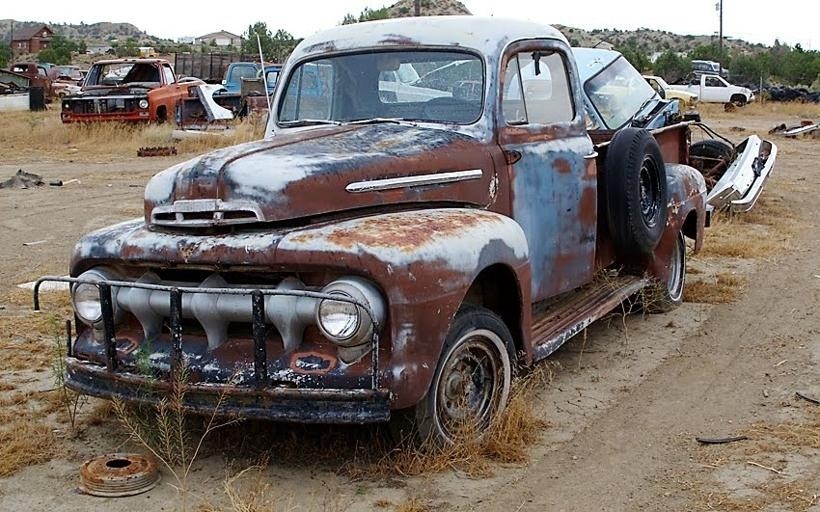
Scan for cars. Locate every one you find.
[0,46,539,123]
[593,75,700,110]
[690,60,728,81]
[32,11,715,459]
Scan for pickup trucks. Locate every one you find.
[664,73,756,108]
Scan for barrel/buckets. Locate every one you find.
[29,87,44,110]
